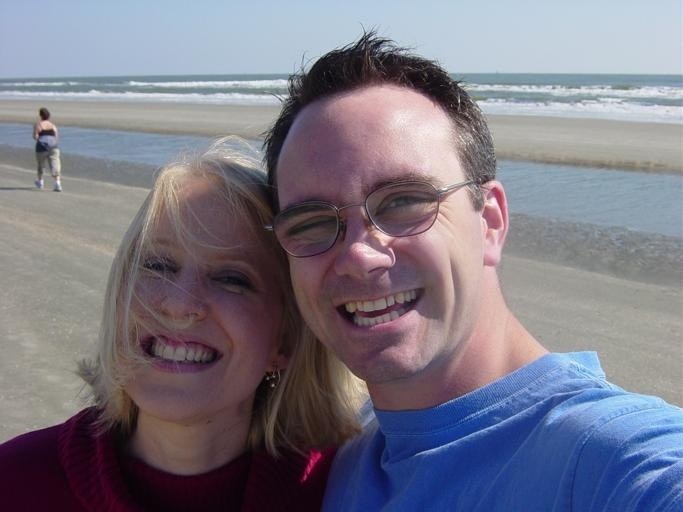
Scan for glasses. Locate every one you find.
[262,179,498,259]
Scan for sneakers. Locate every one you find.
[34,179,43,188]
[53,185,62,191]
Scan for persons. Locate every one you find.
[261,22,681,510]
[31,106,62,192]
[0,135,371,510]
